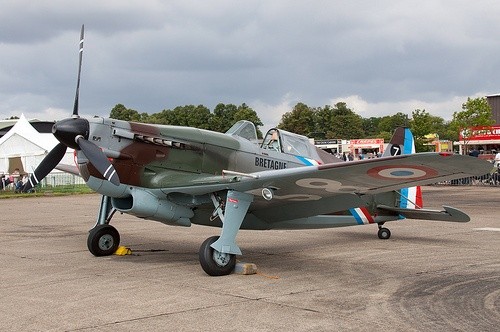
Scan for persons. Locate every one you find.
[0,168,20,190]
[330,151,382,161]
[16,172,28,189]
[469,147,478,157]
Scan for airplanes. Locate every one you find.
[20,23,497,276]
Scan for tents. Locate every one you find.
[0,113,66,174]
